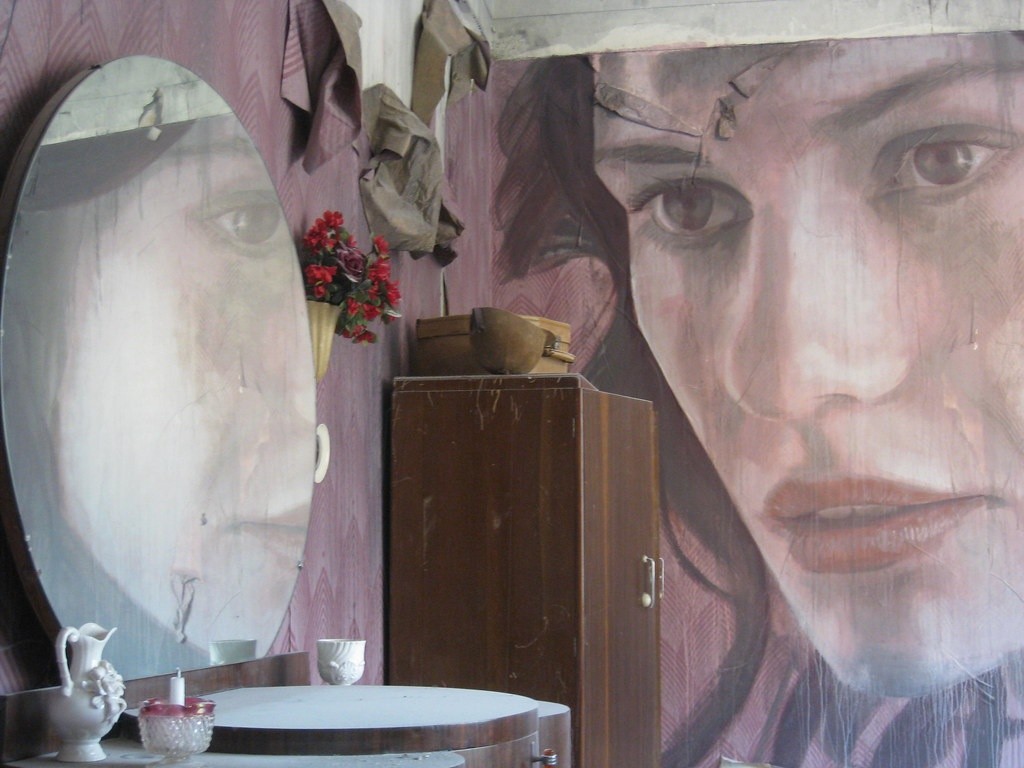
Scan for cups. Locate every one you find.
[138,697,216,766]
[316,638,366,686]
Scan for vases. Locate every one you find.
[306,301,343,380]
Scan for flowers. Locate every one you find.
[298,210,403,344]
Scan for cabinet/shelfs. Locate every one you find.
[389,376,663,768]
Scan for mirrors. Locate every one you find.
[0,55,316,681]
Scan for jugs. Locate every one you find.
[46,623,127,763]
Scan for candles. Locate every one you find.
[169,668,185,706]
